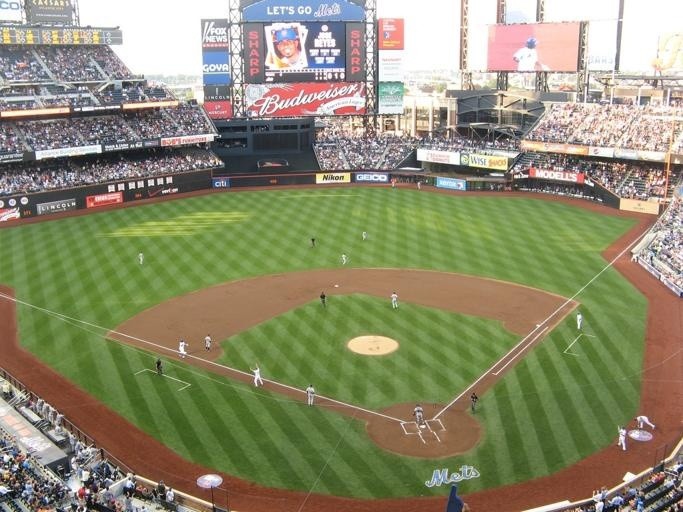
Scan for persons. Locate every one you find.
[616,424,626,452]
[155,357,163,375]
[0,375,174,512]
[313,127,520,191]
[137,251,142,264]
[272,28,301,67]
[311,238,315,247]
[563,451,682,512]
[203,333,212,353]
[411,402,424,425]
[340,253,346,264]
[178,340,188,359]
[390,292,398,311]
[0,44,226,218]
[250,363,263,388]
[447,485,470,512]
[304,384,316,408]
[513,36,548,70]
[519,65,682,296]
[318,290,325,306]
[576,311,582,330]
[361,231,367,241]
[633,416,655,431]
[470,393,477,411]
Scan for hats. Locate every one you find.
[272,28,299,42]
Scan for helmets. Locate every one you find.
[526,38,537,46]
